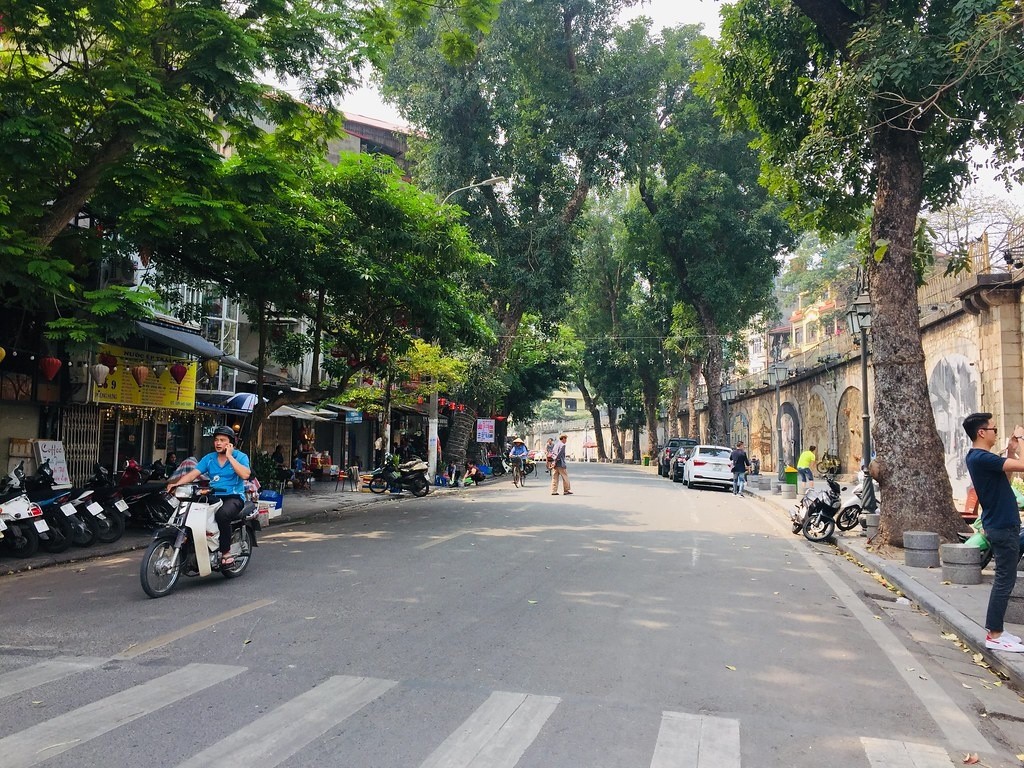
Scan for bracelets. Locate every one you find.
[1016,436,1024,440]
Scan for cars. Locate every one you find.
[682,445,734,489]
[669,445,694,482]
[528,450,547,461]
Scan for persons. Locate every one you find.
[167,426,251,565]
[446,461,459,480]
[730,440,750,498]
[166,452,177,470]
[545,438,555,473]
[797,446,816,488]
[962,413,1024,652]
[550,434,573,495]
[509,438,528,484]
[464,462,483,485]
[271,445,285,464]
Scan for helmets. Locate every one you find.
[213,425,235,441]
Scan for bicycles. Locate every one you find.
[509,454,525,488]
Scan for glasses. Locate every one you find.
[981,428,997,433]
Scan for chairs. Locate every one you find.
[335,467,357,491]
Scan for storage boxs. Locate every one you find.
[257,500,277,519]
[322,465,339,476]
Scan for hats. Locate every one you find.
[513,438,524,443]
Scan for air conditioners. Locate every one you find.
[106,255,138,287]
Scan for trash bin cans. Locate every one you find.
[785,465,798,493]
[644,455,649,466]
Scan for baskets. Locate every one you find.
[547,457,554,462]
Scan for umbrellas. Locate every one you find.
[227,392,268,438]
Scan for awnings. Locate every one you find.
[111,315,290,382]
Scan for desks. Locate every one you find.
[294,471,314,489]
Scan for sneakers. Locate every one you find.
[1002,630,1022,643]
[985,634,1024,652]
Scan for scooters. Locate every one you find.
[488,451,534,477]
[963,485,1024,570]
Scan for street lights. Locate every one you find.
[584,420,589,462]
[719,380,736,448]
[659,409,668,446]
[766,361,789,491]
[845,285,871,536]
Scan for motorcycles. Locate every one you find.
[369,452,433,497]
[837,466,881,531]
[0,458,173,559]
[790,474,847,542]
[140,484,262,598]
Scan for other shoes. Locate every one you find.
[521,471,524,475]
[552,493,559,495]
[545,469,549,473]
[564,492,572,495]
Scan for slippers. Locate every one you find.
[221,556,234,566]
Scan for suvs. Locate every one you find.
[658,438,699,477]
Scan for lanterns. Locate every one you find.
[91,363,110,406]
[169,364,187,400]
[0,347,6,361]
[132,365,148,388]
[203,359,218,395]
[41,355,62,404]
